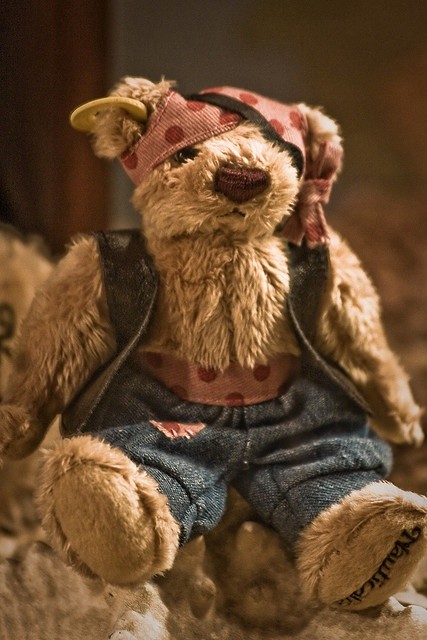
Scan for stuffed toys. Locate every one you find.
[1,76,427,613]
[2,224,427,639]
